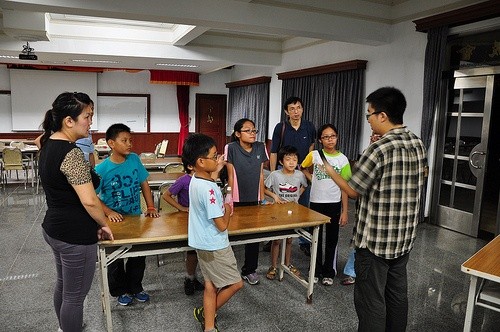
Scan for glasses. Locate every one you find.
[321,135,337,140]
[366,111,389,120]
[241,129,257,134]
[197,152,218,166]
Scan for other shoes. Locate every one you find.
[308,276,319,283]
[287,264,301,277]
[267,266,278,279]
[322,278,333,285]
[202,326,220,332]
[194,306,218,326]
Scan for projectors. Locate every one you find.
[19,54,38,60]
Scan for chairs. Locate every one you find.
[157,182,178,266]
[139,189,155,214]
[0,147,31,190]
[10,141,38,184]
[163,160,185,173]
[0,139,10,190]
[156,139,169,158]
[141,153,157,159]
[96,137,108,145]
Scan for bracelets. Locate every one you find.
[147,207,155,209]
[262,199,267,204]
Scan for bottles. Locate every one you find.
[225,187,234,216]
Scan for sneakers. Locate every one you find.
[134,291,150,302]
[118,294,133,306]
[192,276,204,291]
[184,276,195,296]
[242,273,259,285]
[342,274,355,284]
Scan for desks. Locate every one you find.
[139,172,225,187]
[96,202,330,332]
[459,235,500,332]
[0,138,43,194]
[94,157,185,169]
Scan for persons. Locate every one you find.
[325,87,430,332]
[162,157,206,296]
[263,96,316,256]
[263,145,308,281]
[223,118,274,285]
[92,124,160,305]
[38,92,113,332]
[300,124,351,284]
[182,133,246,332]
[344,132,382,284]
